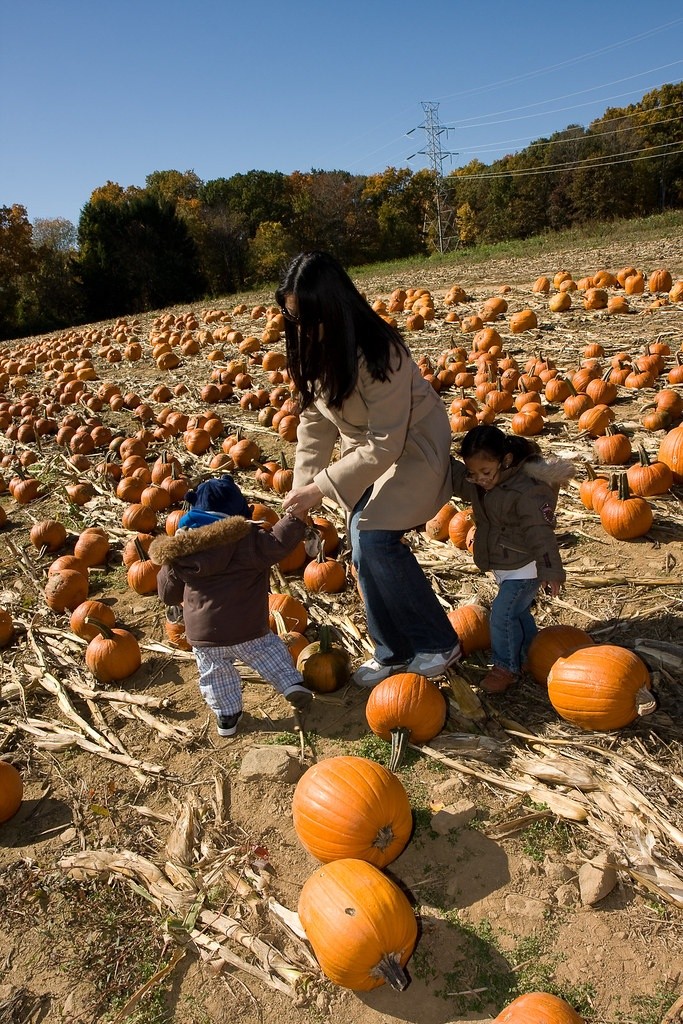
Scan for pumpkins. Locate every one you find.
[296,858,419,994]
[360,268,683,772]
[292,754,413,869]
[0,305,349,691]
[491,992,584,1024]
[0,761,23,823]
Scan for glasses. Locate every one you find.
[463,462,501,485]
[281,309,300,324]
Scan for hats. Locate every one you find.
[186,475,250,518]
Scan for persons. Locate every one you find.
[275,251,463,690]
[453,425,572,694]
[148,474,313,737]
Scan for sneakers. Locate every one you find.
[284,684,314,708]
[480,666,519,693]
[353,658,419,687]
[217,710,244,736]
[407,643,462,678]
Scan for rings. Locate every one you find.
[289,506,295,513]
